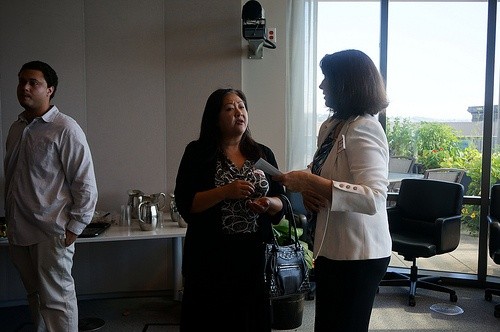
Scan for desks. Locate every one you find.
[0,212,188,302]
[388,173,424,181]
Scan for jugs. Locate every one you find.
[169,193,180,222]
[127,189,145,220]
[145,191,166,224]
[137,201,159,231]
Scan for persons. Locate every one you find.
[5,61,98,332]
[272,50,392,332]
[174,88,287,332]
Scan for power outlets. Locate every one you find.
[268,27,276,42]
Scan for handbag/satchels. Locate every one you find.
[256,193,315,306]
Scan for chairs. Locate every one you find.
[376,179,464,307]
[285,187,316,300]
[390,156,464,183]
[484,184,500,317]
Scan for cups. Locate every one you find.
[179,215,188,228]
[119,205,130,226]
